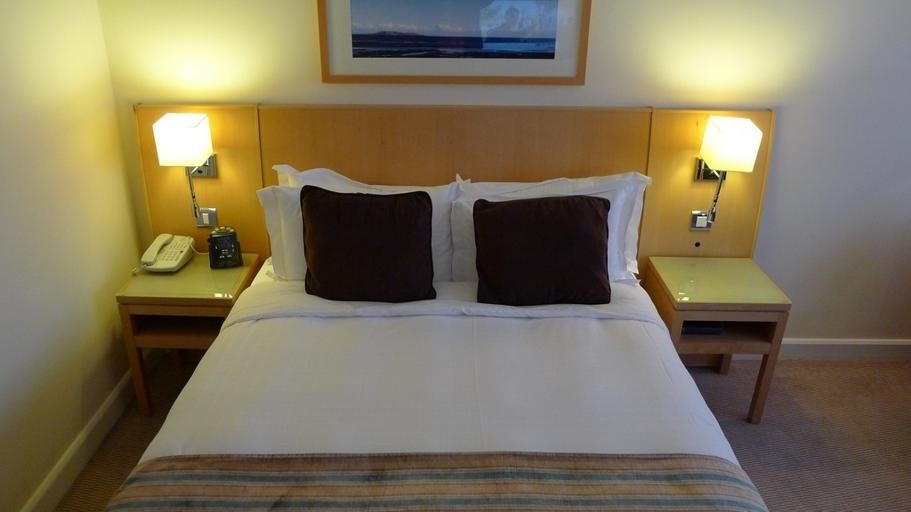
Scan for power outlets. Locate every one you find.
[689,211,714,231]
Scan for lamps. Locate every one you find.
[152,113,215,224]
[694,114,763,227]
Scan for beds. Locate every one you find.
[104,104,770,511]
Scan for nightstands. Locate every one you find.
[115,250,259,418]
[645,253,791,427]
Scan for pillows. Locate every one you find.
[255,181,455,281]
[271,163,473,198]
[450,173,650,287]
[471,195,615,306]
[299,183,438,306]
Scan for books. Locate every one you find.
[683,323,721,335]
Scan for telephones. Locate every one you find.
[140,234,195,273]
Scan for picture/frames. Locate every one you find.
[317,0,593,87]
